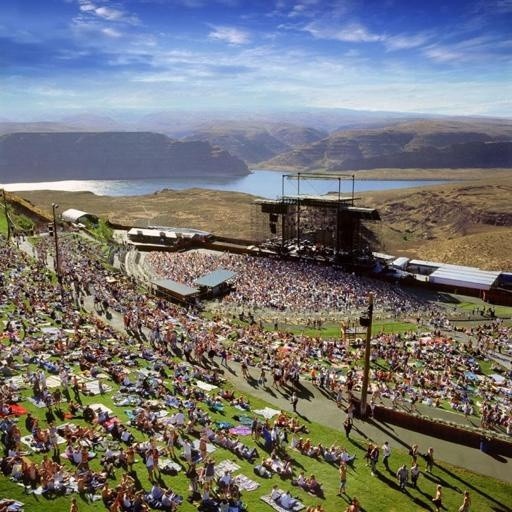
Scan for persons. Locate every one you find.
[1,225,511,512]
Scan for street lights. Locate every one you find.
[359,291,373,415]
[48,203,59,271]
[0,189,7,209]
